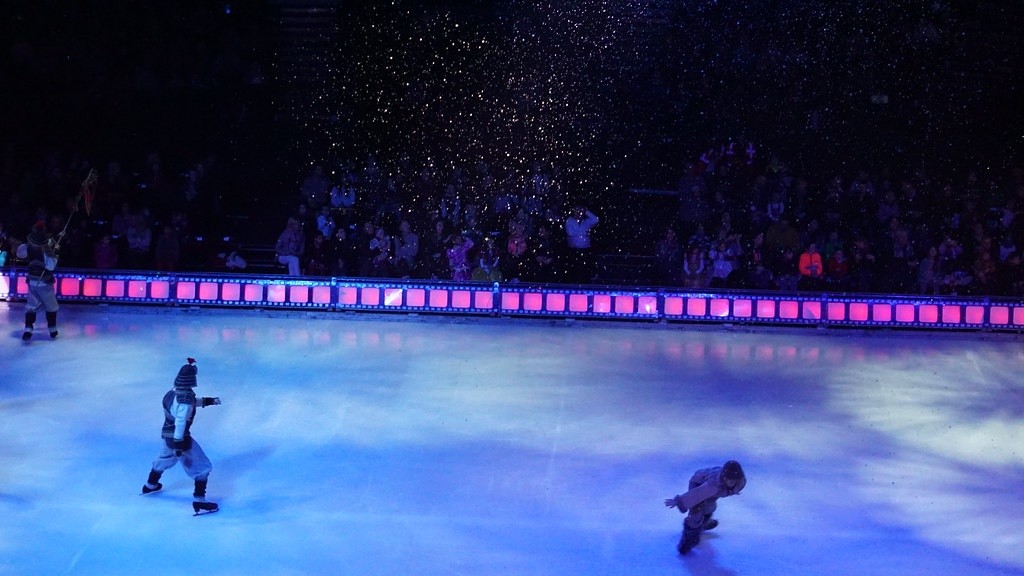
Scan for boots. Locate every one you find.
[44,311,59,341]
[22,312,36,343]
[193,479,219,516]
[138,466,164,496]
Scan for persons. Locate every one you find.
[141,363,221,518]
[273,142,602,284]
[20,220,59,339]
[651,125,1023,295]
[665,460,748,554]
[0,161,248,270]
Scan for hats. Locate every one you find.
[174,357,198,389]
[719,460,745,480]
[26,220,50,248]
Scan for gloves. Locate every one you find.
[174,439,184,458]
[202,396,221,409]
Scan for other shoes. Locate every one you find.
[677,514,719,555]
